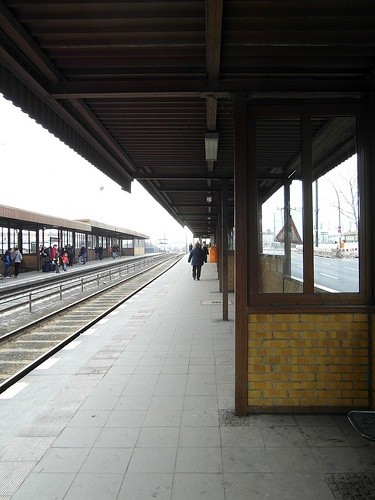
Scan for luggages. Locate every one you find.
[42,264,49,272]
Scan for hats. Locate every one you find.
[64,253,68,255]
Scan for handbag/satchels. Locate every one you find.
[1,255,7,262]
[48,261,55,271]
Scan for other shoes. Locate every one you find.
[6,276,11,278]
[15,275,19,278]
[56,271,60,273]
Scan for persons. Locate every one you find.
[11,248,23,278]
[1,248,12,278]
[40,245,76,273]
[78,247,87,265]
[95,246,103,260]
[189,241,209,263]
[112,244,121,259]
[188,243,207,280]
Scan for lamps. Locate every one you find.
[207,206,212,232]
[204,130,219,162]
[206,194,213,203]
